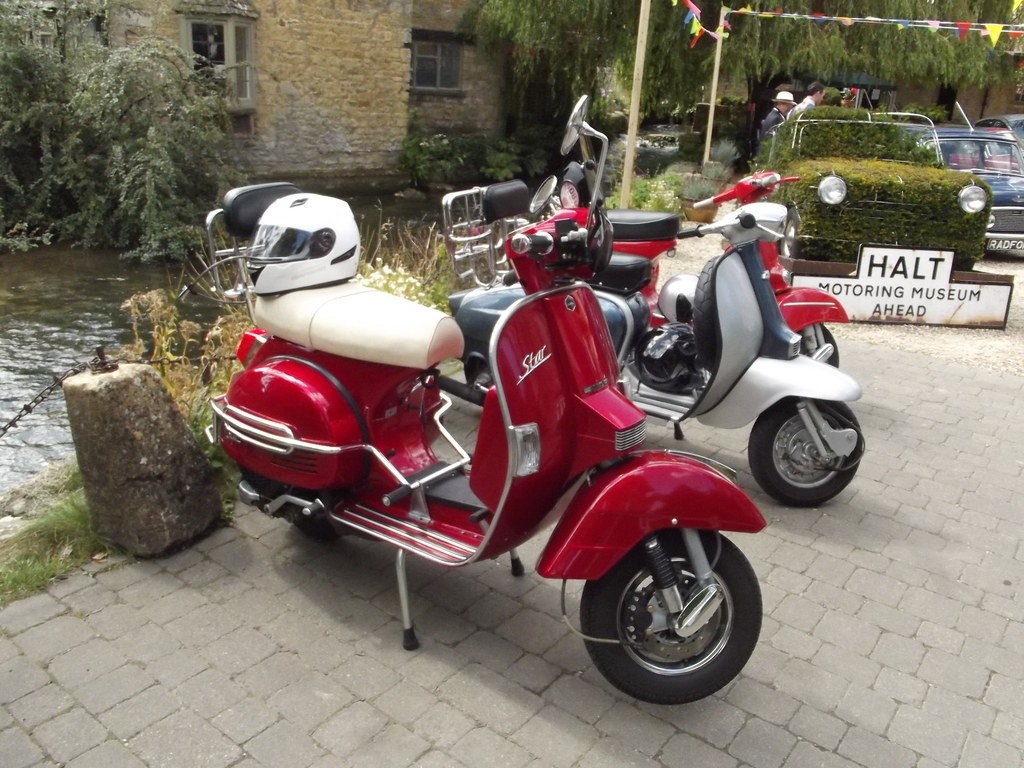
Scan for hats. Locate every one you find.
[771,91,797,106]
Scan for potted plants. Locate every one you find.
[701,160,733,192]
[680,164,728,223]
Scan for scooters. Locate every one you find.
[200,93,769,706]
[440,179,866,509]
[598,156,852,371]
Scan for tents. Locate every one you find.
[801,69,900,117]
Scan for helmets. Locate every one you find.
[631,322,705,394]
[659,272,697,322]
[245,192,362,295]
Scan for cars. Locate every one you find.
[948,114,1024,172]
[899,125,1023,251]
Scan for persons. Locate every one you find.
[760,81,826,144]
